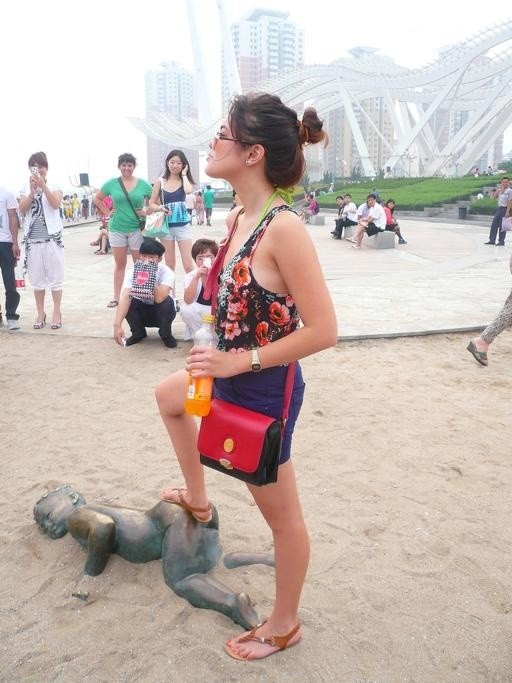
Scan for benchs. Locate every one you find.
[309,213,325,225]
[343,225,396,249]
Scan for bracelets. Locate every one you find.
[181,173,187,177]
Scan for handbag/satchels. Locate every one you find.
[500,216,512,233]
[141,210,170,238]
[129,259,158,306]
[138,221,200,230]
[197,397,283,488]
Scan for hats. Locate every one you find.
[139,240,166,256]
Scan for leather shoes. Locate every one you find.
[157,328,178,349]
[346,237,358,243]
[125,332,148,346]
[351,245,361,249]
[331,230,341,239]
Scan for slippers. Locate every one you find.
[95,248,106,255]
[90,240,99,246]
[107,300,118,307]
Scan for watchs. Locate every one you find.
[250,348,263,374]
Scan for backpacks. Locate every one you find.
[311,201,320,216]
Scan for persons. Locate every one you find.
[476,190,484,199]
[93,152,162,308]
[59,191,112,223]
[300,184,408,249]
[0,183,22,332]
[112,238,178,349]
[233,187,242,207]
[146,148,197,312]
[177,238,220,343]
[14,149,65,330]
[202,184,214,227]
[486,164,493,174]
[482,176,512,246]
[195,190,205,225]
[89,214,109,255]
[465,250,511,366]
[470,167,480,177]
[152,89,339,666]
[491,188,498,198]
[33,482,278,631]
[483,190,490,199]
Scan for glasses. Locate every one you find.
[213,128,255,146]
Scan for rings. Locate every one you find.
[38,174,41,177]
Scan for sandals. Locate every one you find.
[466,340,489,367]
[50,312,61,329]
[223,618,303,661]
[160,487,214,524]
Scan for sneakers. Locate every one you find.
[496,240,505,246]
[484,241,495,245]
[7,319,21,330]
[197,220,211,226]
[398,238,407,244]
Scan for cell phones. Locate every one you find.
[31,167,40,179]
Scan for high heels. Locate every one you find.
[33,312,46,329]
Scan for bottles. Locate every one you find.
[183,314,216,418]
